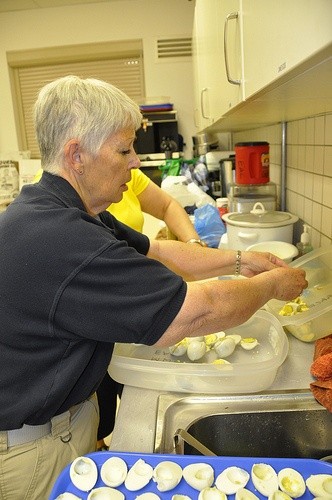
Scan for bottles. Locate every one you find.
[165,143,171,161]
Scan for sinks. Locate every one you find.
[152,388,332,467]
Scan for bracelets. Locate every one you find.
[233,250,241,277]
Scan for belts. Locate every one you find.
[6,404,79,448]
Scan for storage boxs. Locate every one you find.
[142,110,178,120]
[107,309,291,397]
[263,245,332,343]
[132,120,180,156]
[139,103,173,112]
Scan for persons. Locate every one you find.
[0,75,308,500]
[25,166,208,453]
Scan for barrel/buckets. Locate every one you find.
[234,142,271,185]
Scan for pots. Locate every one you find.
[221,201,299,250]
[192,132,220,160]
[219,154,236,197]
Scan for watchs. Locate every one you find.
[187,239,208,247]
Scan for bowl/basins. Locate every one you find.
[244,240,299,263]
[107,309,289,395]
[265,244,332,343]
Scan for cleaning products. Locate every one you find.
[294,223,313,260]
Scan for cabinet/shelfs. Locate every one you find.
[188,0,332,135]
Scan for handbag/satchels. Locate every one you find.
[194,205,226,247]
[160,175,216,210]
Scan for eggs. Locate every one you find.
[54,456,332,500]
[168,331,258,371]
[279,297,315,340]
